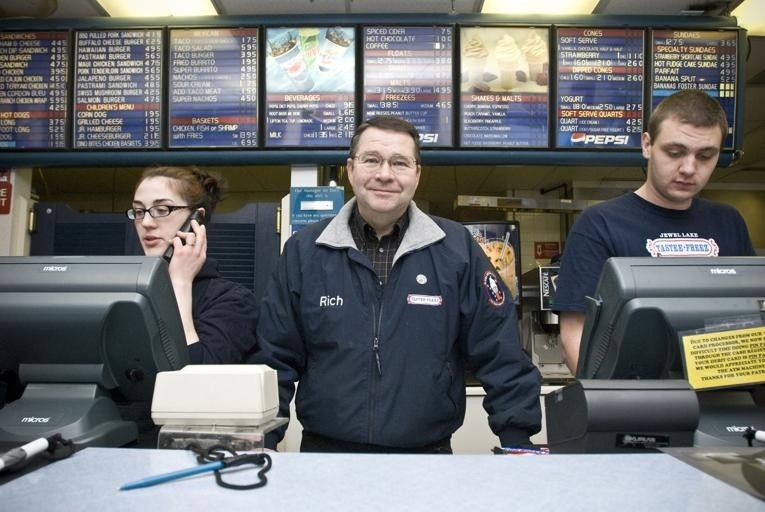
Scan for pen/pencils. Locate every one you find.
[119,460,226,492]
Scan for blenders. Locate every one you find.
[522,267,566,386]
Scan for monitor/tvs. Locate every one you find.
[574,256,761,451]
[2,256,193,449]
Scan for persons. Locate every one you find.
[129,165,259,369]
[250,115,545,456]
[551,91,757,387]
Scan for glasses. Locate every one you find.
[350,151,418,176]
[125,204,191,222]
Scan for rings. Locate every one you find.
[186,243,196,246]
